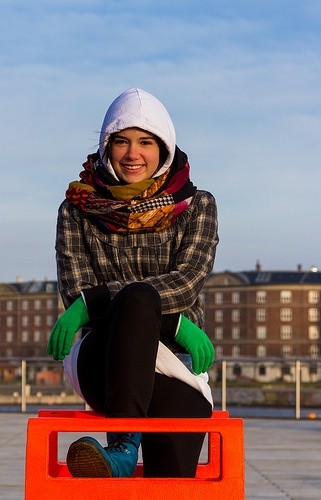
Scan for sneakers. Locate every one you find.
[66,436,138,478]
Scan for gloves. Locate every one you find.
[47,295,89,361]
[174,314,214,375]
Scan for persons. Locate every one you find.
[47,88,219,477]
